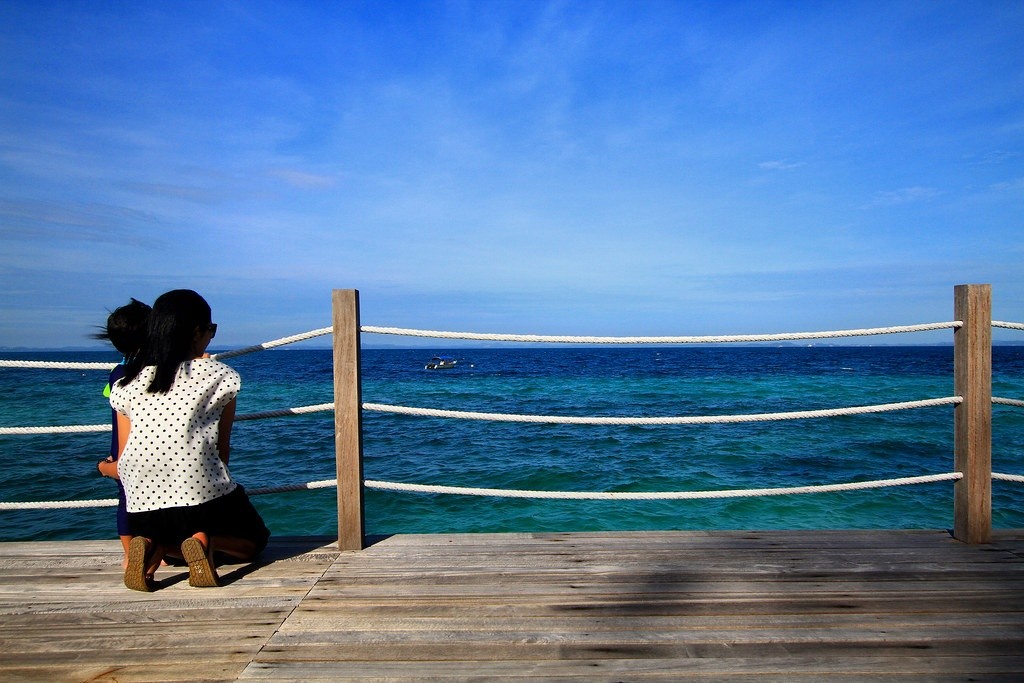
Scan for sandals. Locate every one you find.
[181,532,223,588]
[124,537,156,592]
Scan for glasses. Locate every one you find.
[208,322,218,340]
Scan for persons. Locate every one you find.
[97,289,270,592]
[87,298,210,570]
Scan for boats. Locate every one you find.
[424,356,458,369]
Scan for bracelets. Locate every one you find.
[97,458,112,478]
